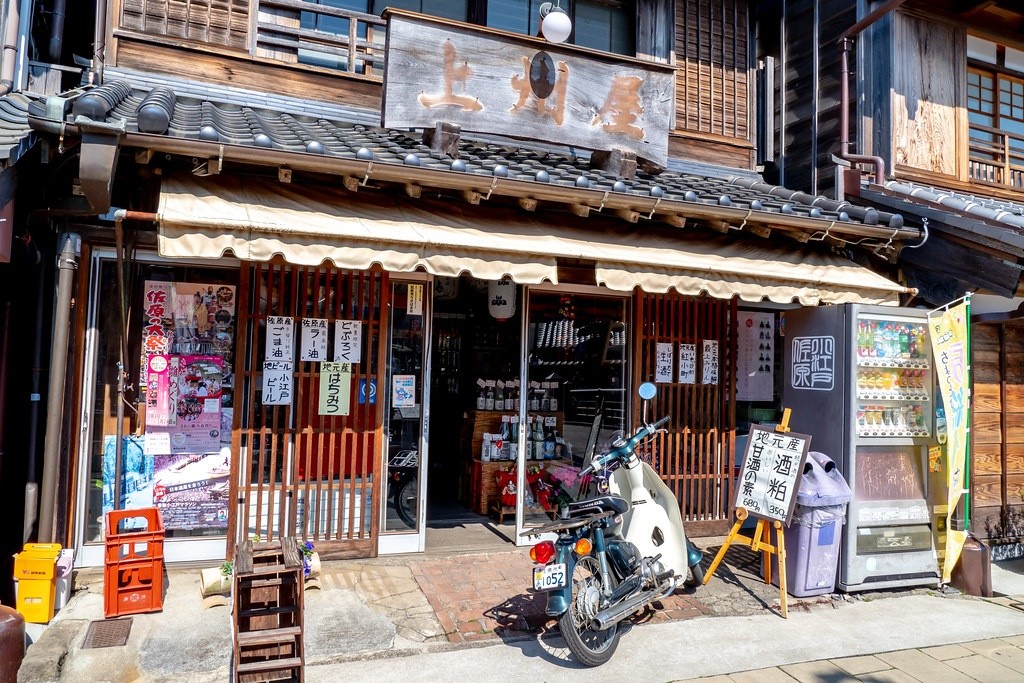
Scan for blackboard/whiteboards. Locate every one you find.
[732,424,812,527]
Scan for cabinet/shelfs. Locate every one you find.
[466,407,575,515]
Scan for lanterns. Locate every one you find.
[487,279,517,321]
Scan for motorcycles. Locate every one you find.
[389,450,417,526]
[518,382,704,669]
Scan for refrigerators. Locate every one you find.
[782,301,946,594]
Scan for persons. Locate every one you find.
[185,380,207,396]
[190,286,231,333]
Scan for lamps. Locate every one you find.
[538,0,571,42]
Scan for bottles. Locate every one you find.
[906,404,916,427]
[860,320,928,391]
[474,379,564,461]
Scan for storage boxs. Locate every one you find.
[102,506,165,618]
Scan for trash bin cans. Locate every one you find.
[760,450,853,597]
